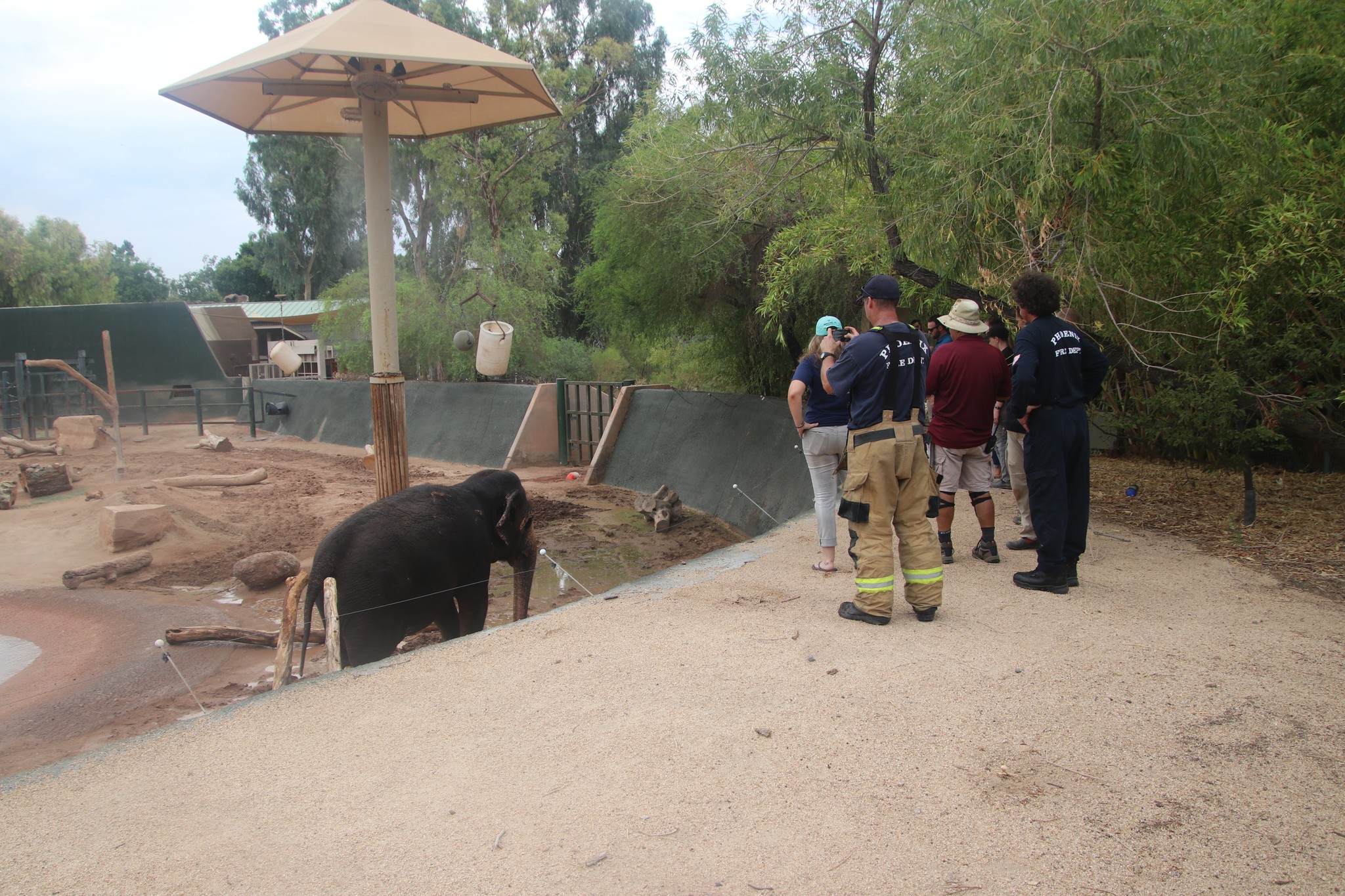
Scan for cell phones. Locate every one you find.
[832,329,851,342]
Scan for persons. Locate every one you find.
[908,313,953,480]
[1009,270,1108,595]
[818,273,944,625]
[1056,304,1081,327]
[926,298,1009,565]
[978,307,1038,551]
[786,315,859,573]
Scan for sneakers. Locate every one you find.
[972,538,1000,563]
[938,538,954,564]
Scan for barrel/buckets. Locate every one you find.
[476,321,514,376]
[269,341,302,374]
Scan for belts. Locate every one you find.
[853,424,924,447]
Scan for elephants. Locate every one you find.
[298,468,536,679]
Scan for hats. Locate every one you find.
[856,273,900,301]
[816,315,842,336]
[937,298,989,333]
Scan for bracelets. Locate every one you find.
[795,421,804,428]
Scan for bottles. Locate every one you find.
[1126,484,1139,497]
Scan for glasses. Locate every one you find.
[928,325,940,332]
[861,286,873,298]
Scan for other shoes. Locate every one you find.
[1006,537,1043,550]
[990,479,1012,489]
[1012,511,1022,524]
[838,602,891,625]
[912,606,938,620]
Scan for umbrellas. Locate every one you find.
[154,0,559,498]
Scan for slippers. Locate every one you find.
[812,561,838,572]
[993,475,1002,479]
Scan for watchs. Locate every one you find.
[994,405,1002,410]
[820,352,835,361]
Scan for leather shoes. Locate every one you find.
[1013,564,1069,594]
[1067,557,1079,586]
